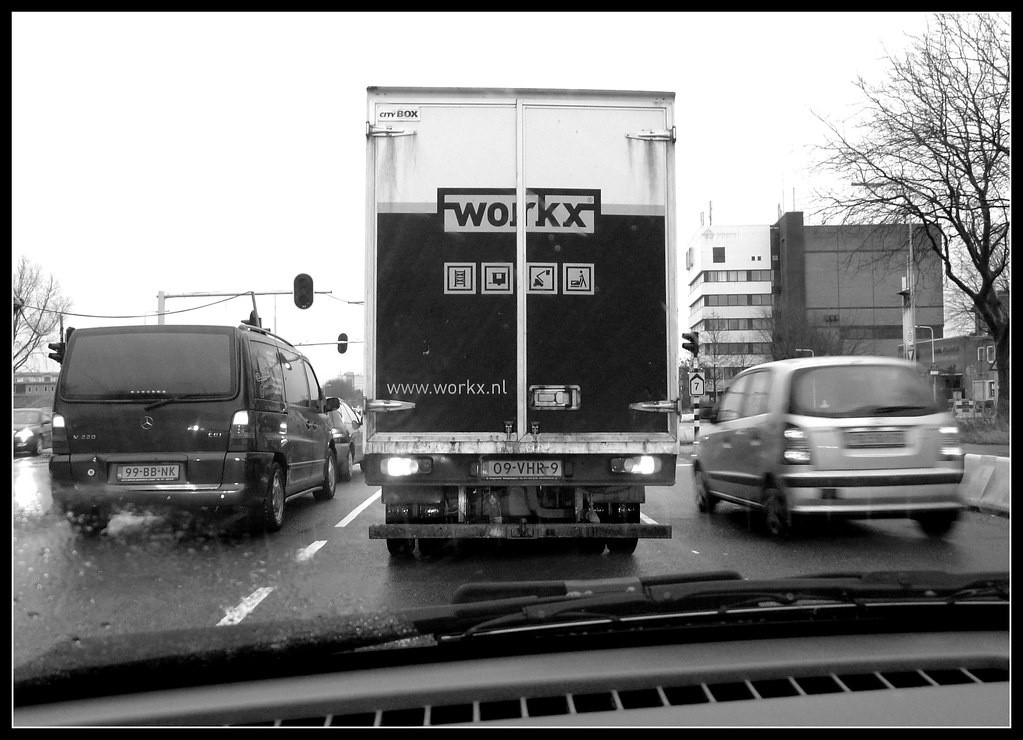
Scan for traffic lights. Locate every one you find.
[681,330,701,356]
[242,311,258,332]
[293,274,314,310]
[48,340,66,366]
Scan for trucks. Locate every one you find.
[359,84,680,562]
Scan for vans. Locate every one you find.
[48,324,342,537]
[691,356,968,541]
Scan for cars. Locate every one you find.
[11,408,54,456]
[324,398,364,483]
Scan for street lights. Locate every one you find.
[915,324,936,404]
[853,181,916,362]
[796,348,816,409]
